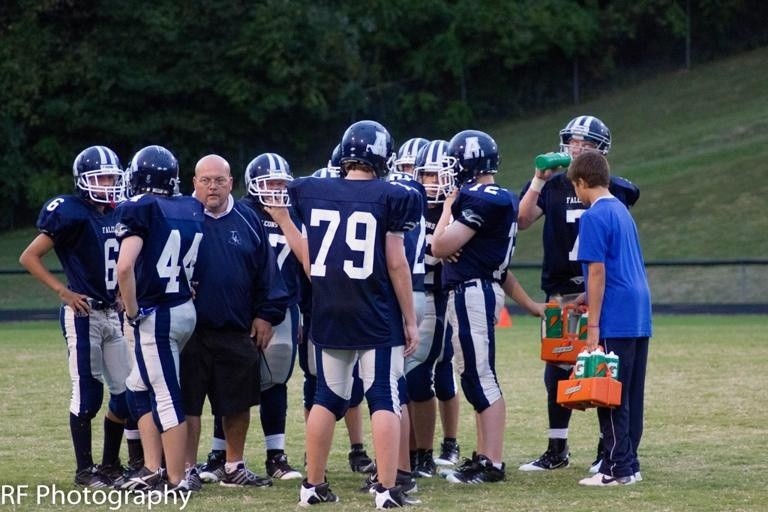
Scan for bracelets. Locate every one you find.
[586,324,600,327]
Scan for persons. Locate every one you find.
[514,113,640,475]
[565,151,654,488]
[16,119,554,509]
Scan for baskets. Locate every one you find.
[541,305,623,411]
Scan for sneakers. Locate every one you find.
[74,442,643,510]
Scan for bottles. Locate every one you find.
[533,150,574,173]
[540,299,588,339]
[573,348,620,378]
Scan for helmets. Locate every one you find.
[73,115,612,206]
